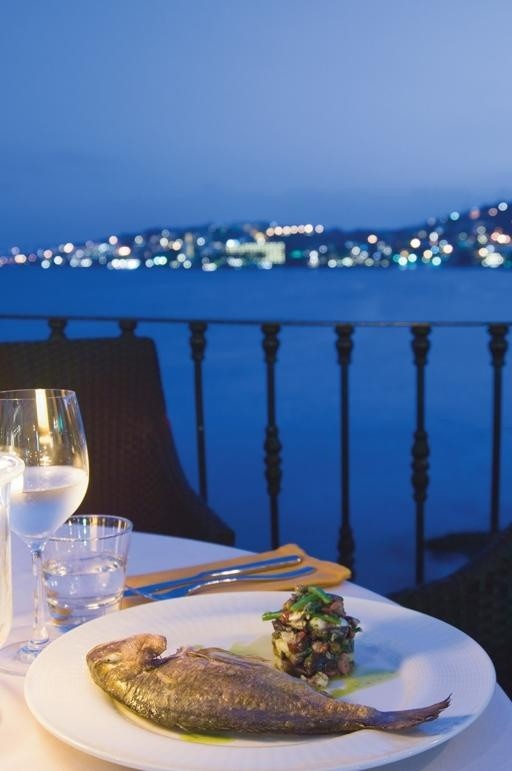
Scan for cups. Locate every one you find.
[39,515,133,633]
[0,389,90,671]
[0,453,27,650]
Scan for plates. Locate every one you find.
[22,591,496,766]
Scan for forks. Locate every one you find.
[131,566,316,602]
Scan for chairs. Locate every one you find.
[388,518,512,696]
[0,337,235,546]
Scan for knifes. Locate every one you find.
[124,553,301,598]
